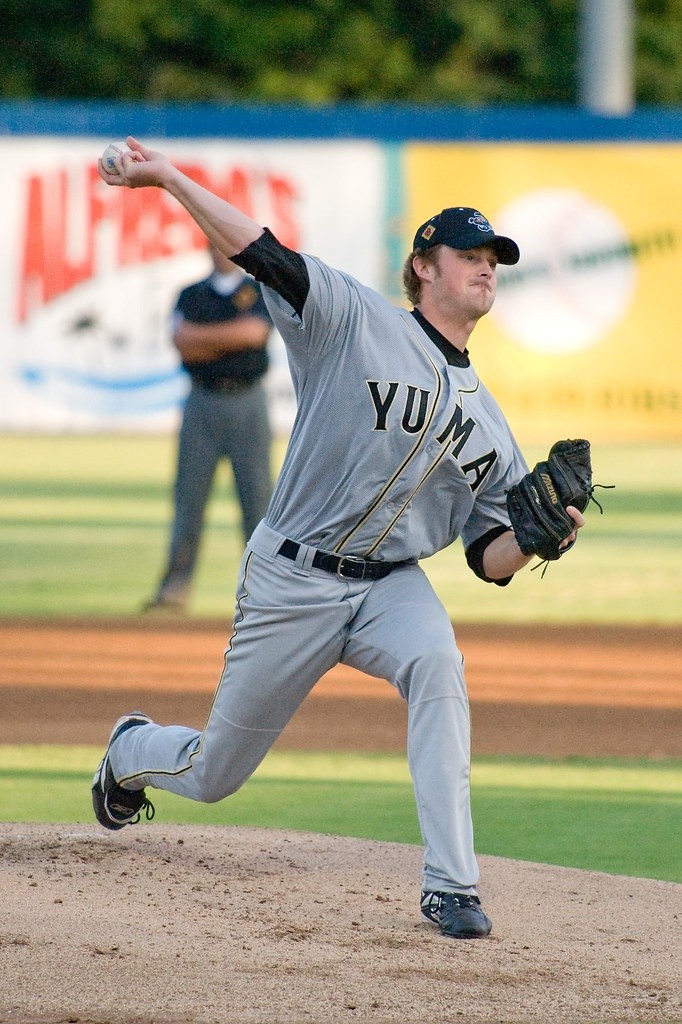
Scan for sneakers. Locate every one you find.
[418,891,493,938]
[91,712,153,830]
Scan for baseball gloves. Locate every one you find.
[505,438,593,561]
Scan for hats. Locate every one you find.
[414,207,521,266]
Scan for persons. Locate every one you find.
[142,238,273,611]
[91,136,595,938]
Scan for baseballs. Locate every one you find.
[101,142,131,175]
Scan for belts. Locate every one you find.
[279,542,394,582]
